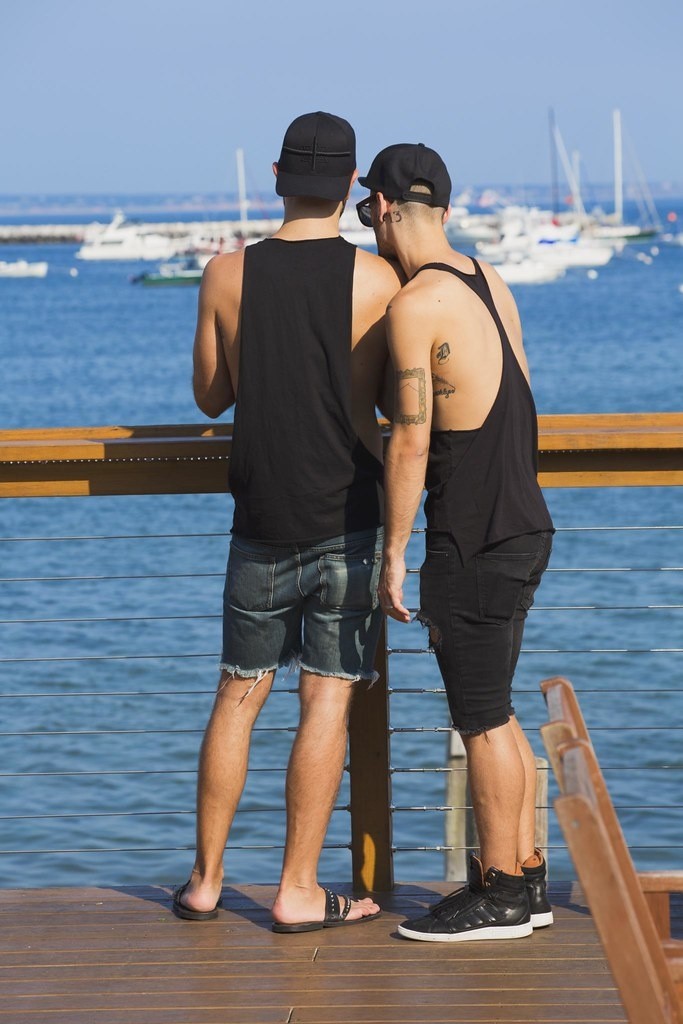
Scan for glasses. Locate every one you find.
[356,194,381,227]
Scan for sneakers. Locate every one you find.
[520,847,554,928]
[397,856,533,942]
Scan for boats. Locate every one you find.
[71,108,660,283]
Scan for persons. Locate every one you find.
[353,141,555,943]
[170,112,404,934]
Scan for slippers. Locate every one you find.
[172,880,219,920]
[272,886,383,932]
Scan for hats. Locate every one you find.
[276,111,357,200]
[357,142,452,208]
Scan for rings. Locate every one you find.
[386,606,393,609]
